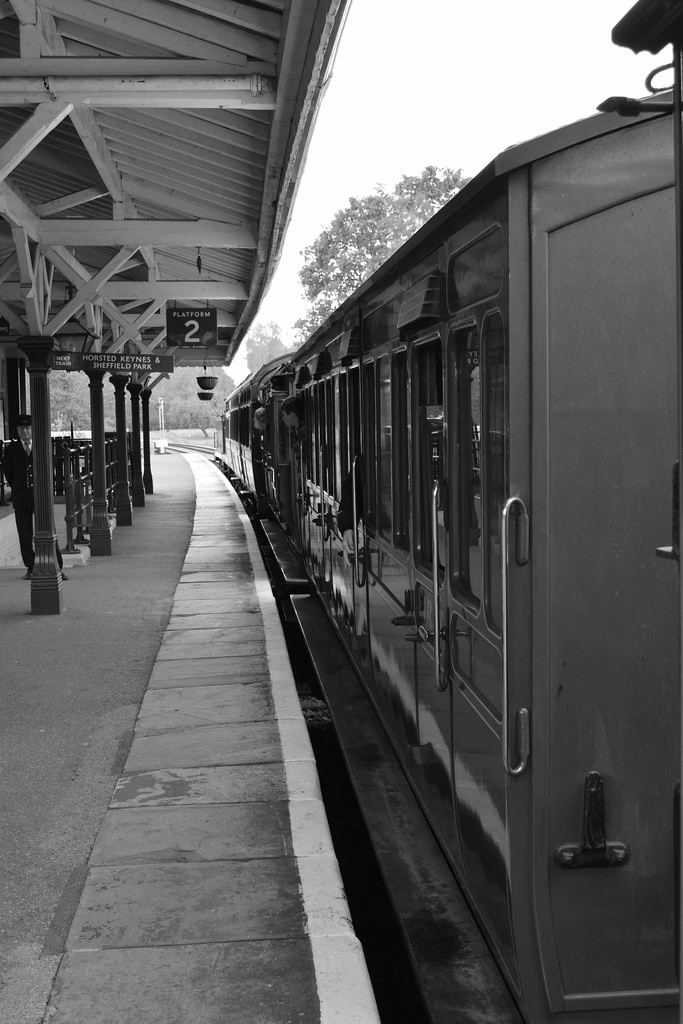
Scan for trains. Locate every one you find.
[215,86,683,1024]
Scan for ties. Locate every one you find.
[24,442,31,456]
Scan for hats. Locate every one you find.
[15,415,32,426]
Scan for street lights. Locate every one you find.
[601,1,683,992]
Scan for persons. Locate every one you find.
[1,415,68,580]
[280,396,304,429]
[253,407,269,430]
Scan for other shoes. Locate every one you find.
[60,572,68,580]
[24,571,33,580]
